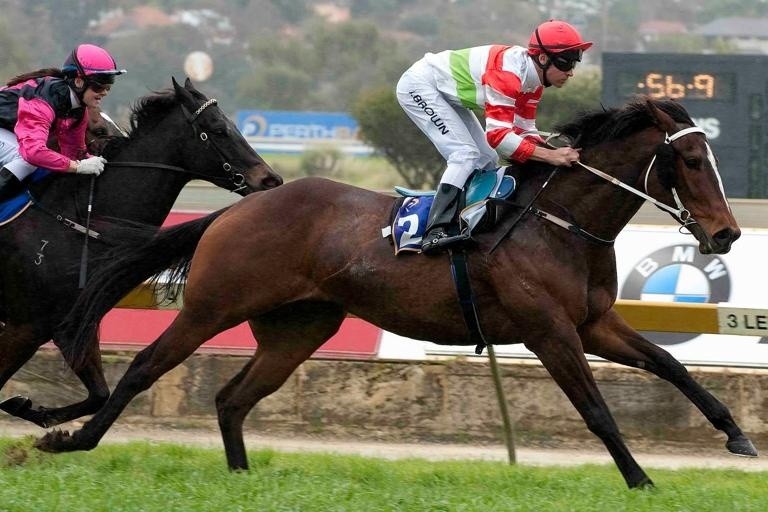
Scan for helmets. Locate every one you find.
[528,19,593,57]
[61,44,127,77]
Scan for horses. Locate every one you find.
[0,77,283,428]
[31,95,759,495]
[88,107,129,155]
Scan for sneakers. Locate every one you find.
[421,182,469,255]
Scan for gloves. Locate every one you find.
[77,155,107,176]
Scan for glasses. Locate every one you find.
[545,52,576,71]
[88,80,111,92]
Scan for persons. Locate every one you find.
[395,19,592,250]
[1,43,125,195]
